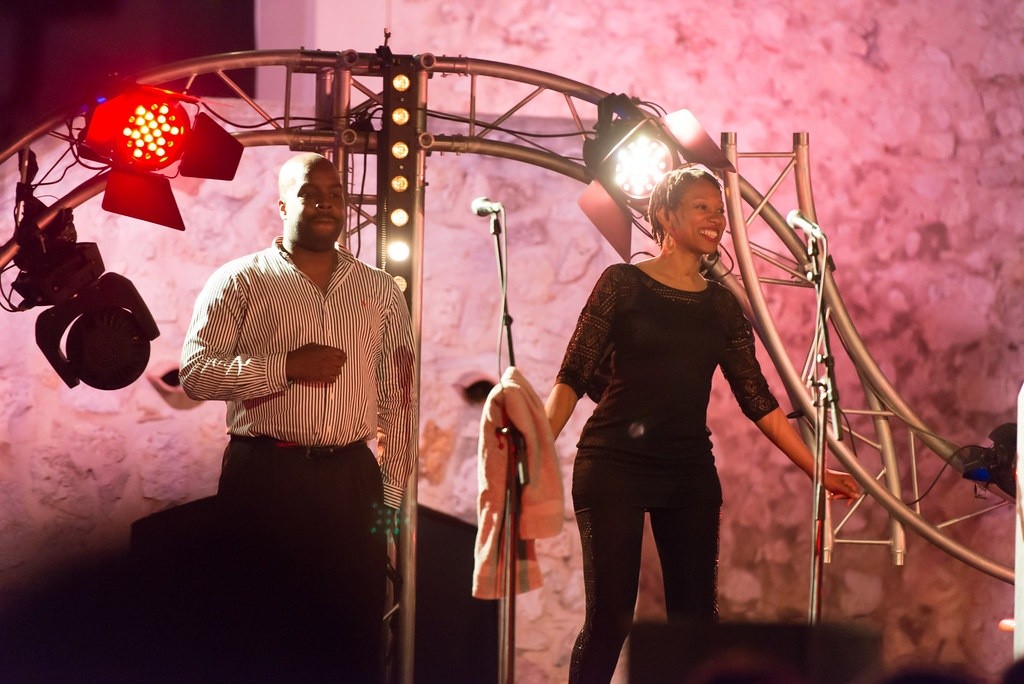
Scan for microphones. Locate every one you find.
[787,209,827,240]
[471,196,502,217]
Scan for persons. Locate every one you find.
[179,153,420,683]
[542,165,861,684]
